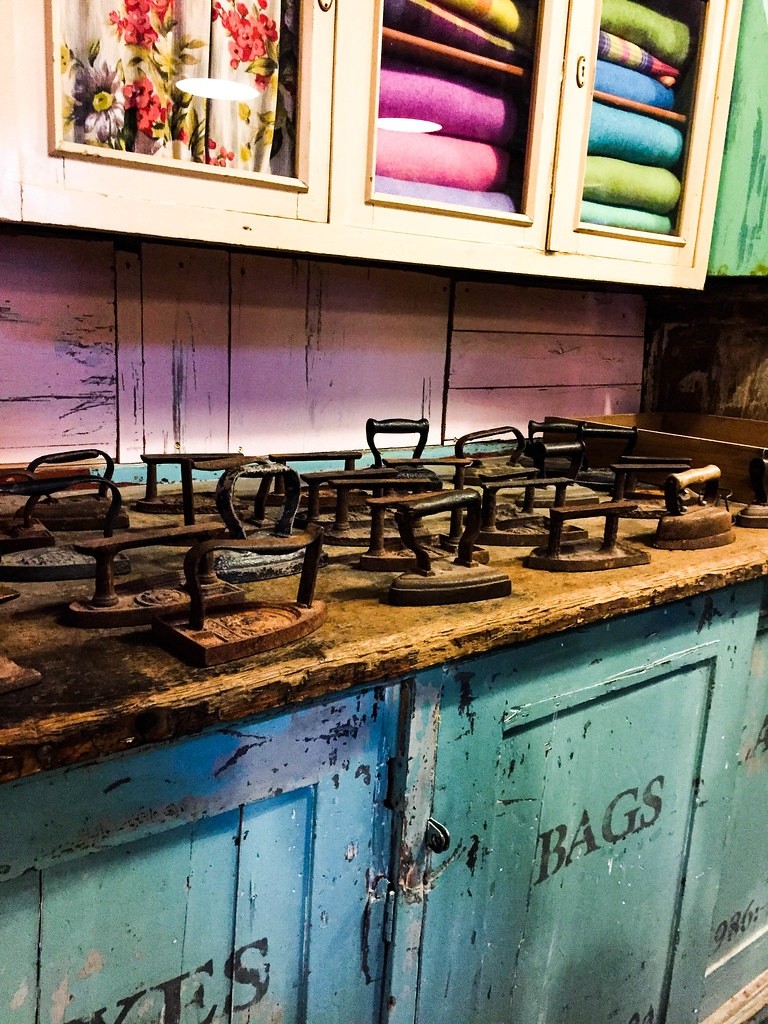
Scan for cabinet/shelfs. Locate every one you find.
[3,574,768,1024]
[0,0,744,292]
